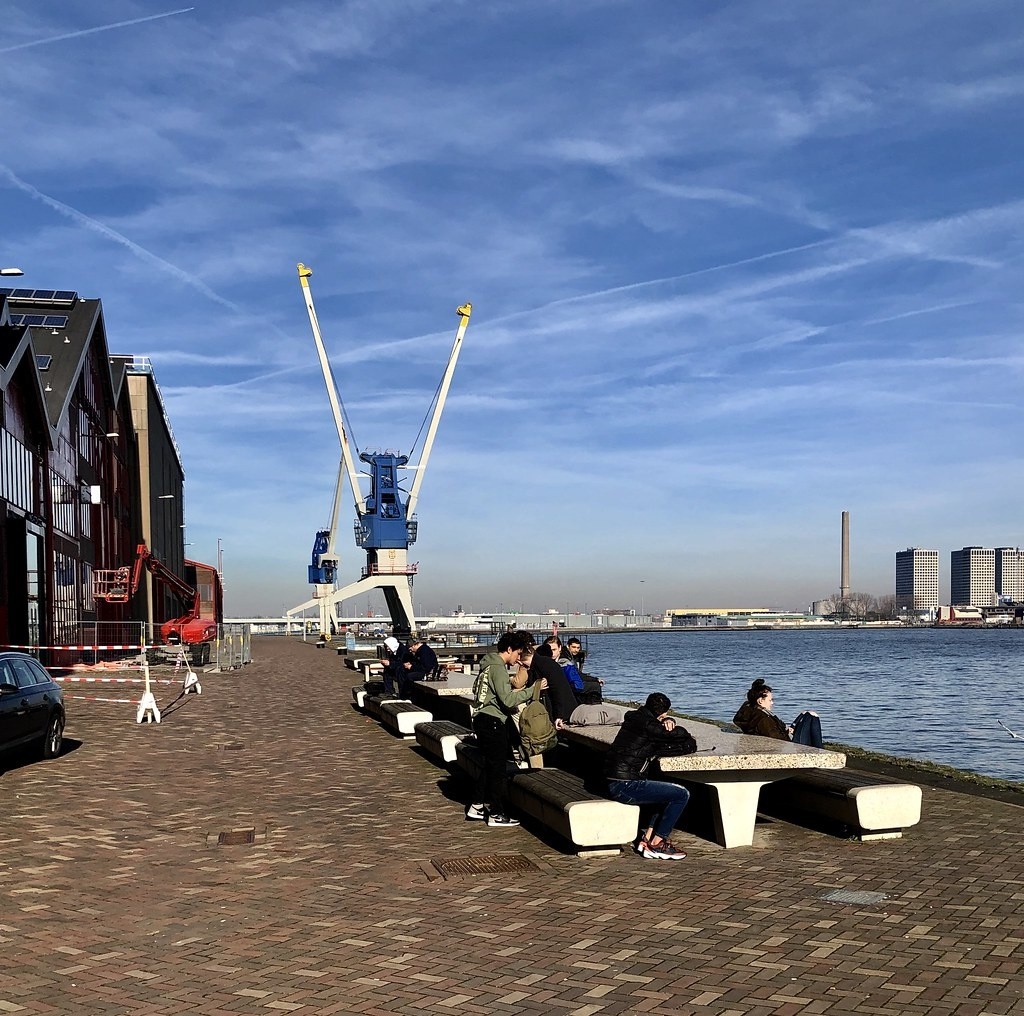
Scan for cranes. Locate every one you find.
[282,261,473,639]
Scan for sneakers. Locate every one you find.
[488,813,520,826]
[467,804,489,820]
[637,835,648,852]
[643,837,687,860]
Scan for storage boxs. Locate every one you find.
[308,565,337,584]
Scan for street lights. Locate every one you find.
[217,538,223,572]
[220,549,224,584]
[640,581,646,615]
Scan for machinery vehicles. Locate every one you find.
[90,543,218,667]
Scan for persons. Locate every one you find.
[466,630,604,827]
[392,637,439,700]
[604,692,689,859]
[379,637,414,696]
[732,678,823,749]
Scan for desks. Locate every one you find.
[378,670,478,714]
[456,693,847,849]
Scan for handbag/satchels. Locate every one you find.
[570,674,604,705]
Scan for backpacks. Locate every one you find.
[427,664,449,681]
[519,679,558,757]
[791,710,825,749]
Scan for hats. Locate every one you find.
[406,639,419,650]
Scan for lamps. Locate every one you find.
[154,494,175,500]
[183,543,196,546]
[173,525,188,528]
[81,432,119,438]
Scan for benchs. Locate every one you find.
[770,770,922,842]
[414,721,476,763]
[514,768,641,859]
[352,685,369,710]
[380,702,432,740]
[367,694,412,722]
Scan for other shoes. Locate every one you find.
[379,692,393,700]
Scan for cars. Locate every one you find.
[0,650,67,761]
